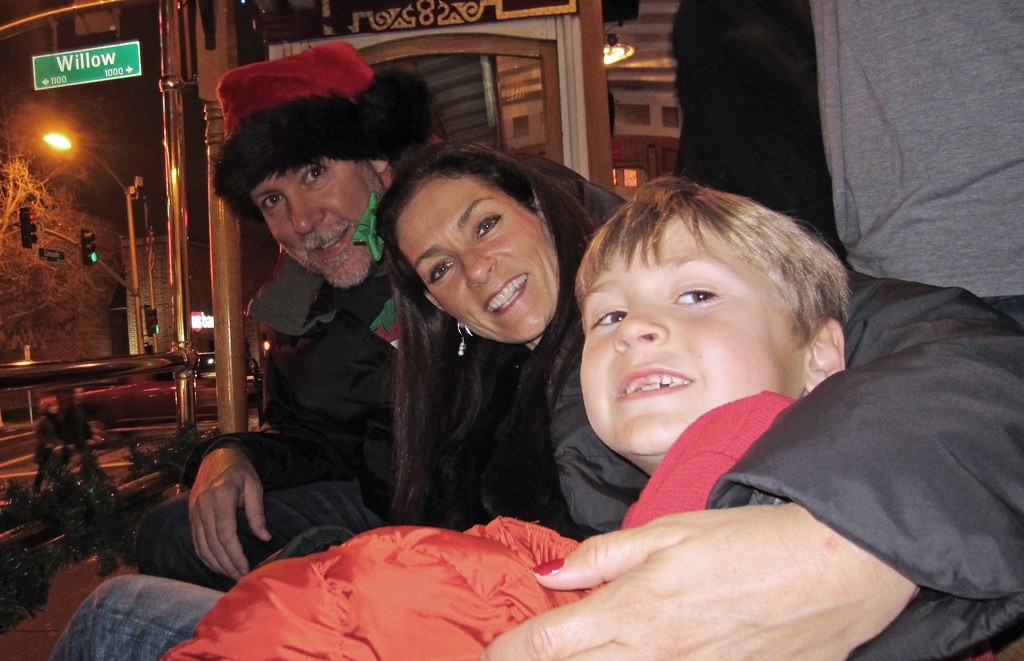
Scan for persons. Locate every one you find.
[32,397,76,494]
[51,0,1024,661]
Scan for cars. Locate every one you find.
[36,346,267,449]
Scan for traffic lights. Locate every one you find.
[144,304,159,337]
[81,227,98,267]
[19,207,38,249]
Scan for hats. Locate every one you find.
[39,396,57,413]
[212,42,429,223]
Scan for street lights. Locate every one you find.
[43,125,145,355]
